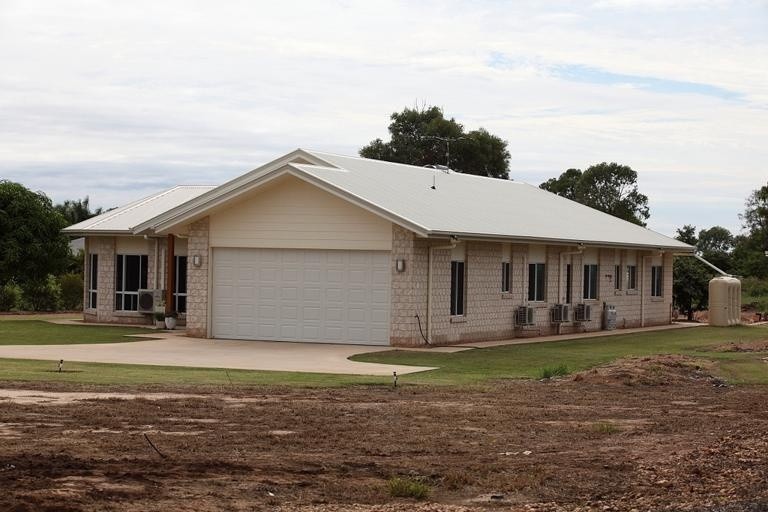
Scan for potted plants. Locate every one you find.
[165,311,179,330]
[155,313,166,328]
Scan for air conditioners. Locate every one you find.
[137,290,166,313]
[576,304,591,321]
[554,304,569,322]
[518,307,533,324]
